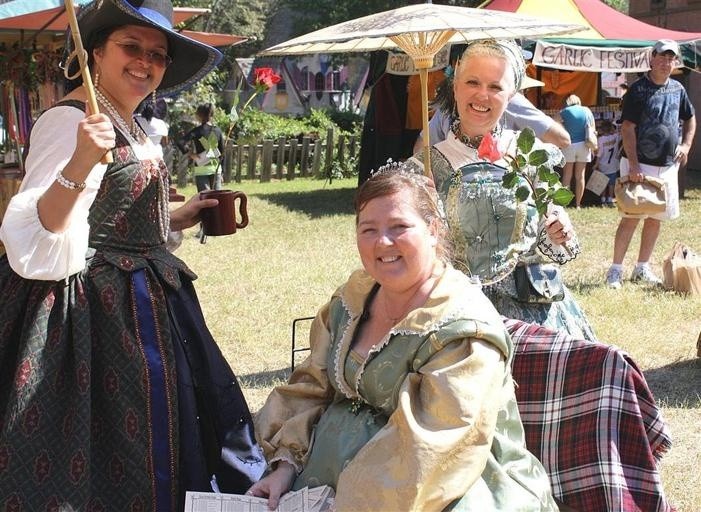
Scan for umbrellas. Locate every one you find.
[256,3,591,176]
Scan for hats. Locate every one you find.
[652,38,678,55]
[66,0,223,104]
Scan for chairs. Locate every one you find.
[497,310,648,512]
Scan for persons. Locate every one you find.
[606,40,697,289]
[414,42,571,157]
[132,101,169,148]
[591,120,623,203]
[406,38,600,350]
[188,102,227,236]
[1,1,253,512]
[241,164,558,512]
[550,94,595,209]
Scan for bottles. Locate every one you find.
[589,105,620,121]
[540,109,560,120]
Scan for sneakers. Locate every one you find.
[606,268,624,291]
[629,267,662,289]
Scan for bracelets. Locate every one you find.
[57,170,86,192]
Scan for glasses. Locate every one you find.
[106,38,172,70]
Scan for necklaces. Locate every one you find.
[81,79,171,244]
[383,283,425,326]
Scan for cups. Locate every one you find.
[199,189,249,236]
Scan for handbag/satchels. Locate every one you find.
[515,263,565,303]
[584,123,598,153]
[614,174,666,216]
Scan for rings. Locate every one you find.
[678,155,682,158]
[247,489,253,495]
[560,230,569,238]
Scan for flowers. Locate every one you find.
[470,126,575,261]
[207,63,283,191]
